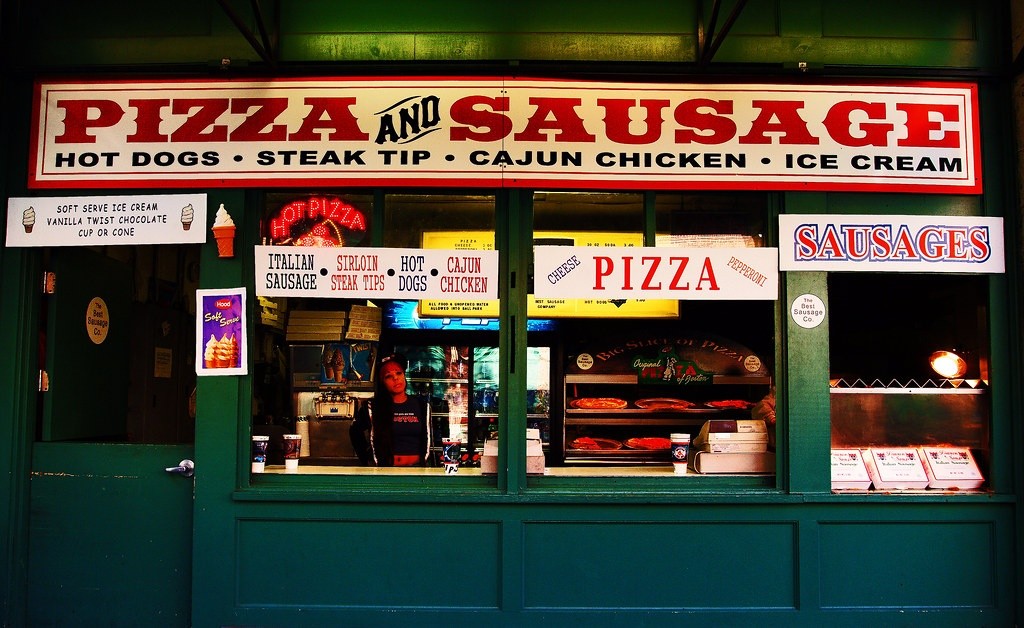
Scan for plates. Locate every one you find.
[570,398,628,409]
[704,399,756,410]
[568,438,622,451]
[634,398,695,409]
[624,437,671,449]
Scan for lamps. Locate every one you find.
[927,348,969,381]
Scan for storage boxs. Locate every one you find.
[831,448,873,489]
[862,449,930,490]
[921,448,986,488]
[287,305,383,343]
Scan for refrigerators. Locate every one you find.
[382,298,556,467]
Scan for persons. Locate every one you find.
[751,381,777,452]
[349,359,433,467]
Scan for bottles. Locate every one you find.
[480,352,489,380]
[406,383,468,414]
[473,384,499,414]
[487,418,497,435]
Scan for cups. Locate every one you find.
[671,433,690,473]
[296,415,310,456]
[442,438,461,476]
[283,434,301,470]
[251,435,269,473]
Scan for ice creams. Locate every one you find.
[322,347,344,382]
[203,333,238,369]
[212,203,236,258]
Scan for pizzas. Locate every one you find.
[576,397,627,409]
[573,437,597,444]
[626,437,672,450]
[710,399,748,408]
